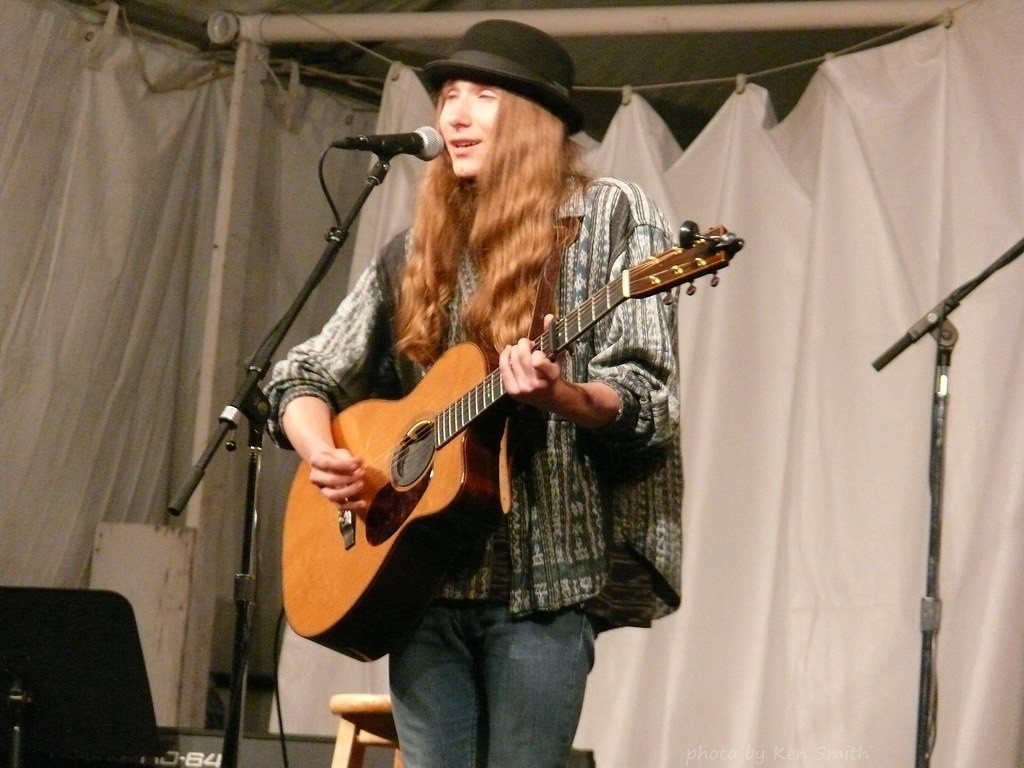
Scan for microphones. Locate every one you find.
[330,125,444,162]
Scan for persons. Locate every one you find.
[261,20,681,768]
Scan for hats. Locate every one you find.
[412,16,587,136]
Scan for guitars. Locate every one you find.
[280,219,746,663]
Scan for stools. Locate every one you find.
[326,691,404,768]
